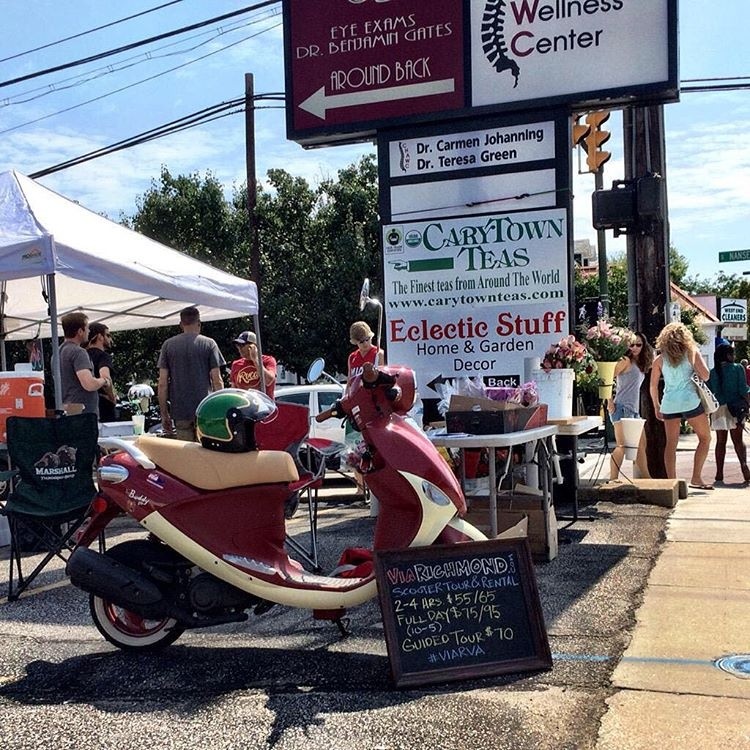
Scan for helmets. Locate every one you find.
[195,389,279,452]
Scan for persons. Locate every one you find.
[157,306,227,442]
[338,321,386,510]
[607,332,654,480]
[50,313,109,446]
[127,373,155,414]
[740,359,750,422]
[86,321,119,422]
[706,343,750,482]
[650,322,716,490]
[230,332,277,403]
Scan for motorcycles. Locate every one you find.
[66,277,491,654]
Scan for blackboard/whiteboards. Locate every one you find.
[374,536,554,687]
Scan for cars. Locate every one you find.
[271,384,347,446]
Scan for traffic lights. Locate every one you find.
[585,111,611,173]
[571,113,591,148]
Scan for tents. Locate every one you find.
[1,170,269,418]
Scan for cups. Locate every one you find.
[132,415,145,435]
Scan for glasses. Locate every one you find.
[629,343,642,348]
[236,343,246,347]
[360,337,371,343]
[107,333,112,338]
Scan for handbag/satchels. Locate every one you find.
[690,373,719,413]
[726,397,749,417]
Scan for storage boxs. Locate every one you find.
[446,404,548,435]
[465,491,558,560]
[98,421,136,436]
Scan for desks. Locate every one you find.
[427,422,558,537]
[544,415,604,521]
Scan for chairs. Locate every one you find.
[0,411,106,601]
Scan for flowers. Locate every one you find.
[539,316,637,398]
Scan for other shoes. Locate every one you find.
[742,481,750,486]
[713,480,724,487]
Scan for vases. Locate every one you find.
[596,360,618,399]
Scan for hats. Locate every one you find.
[233,331,257,344]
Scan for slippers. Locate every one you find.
[689,483,713,490]
